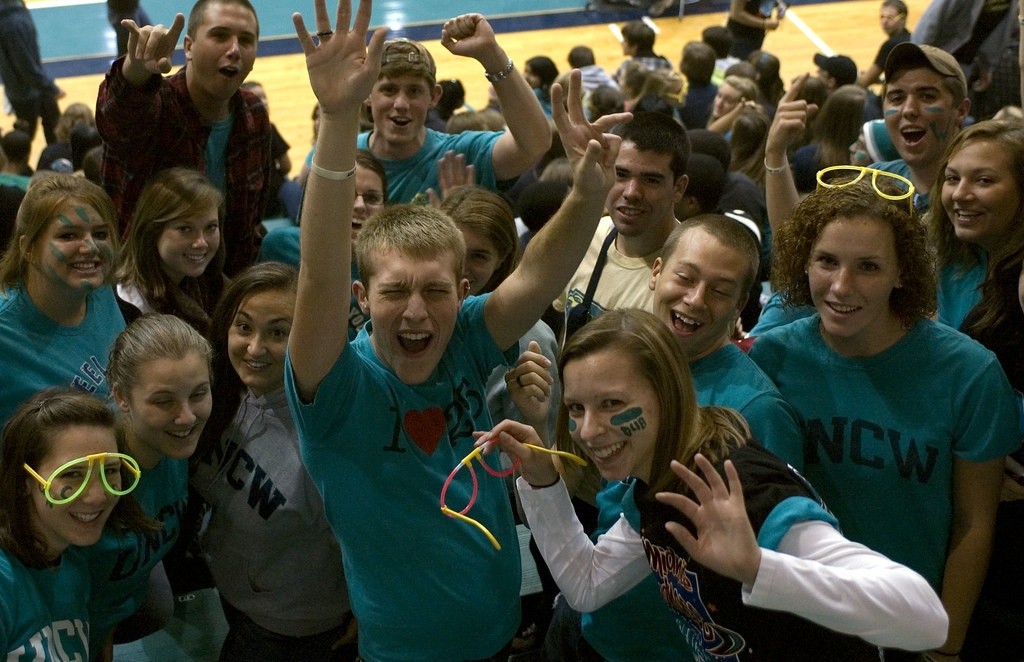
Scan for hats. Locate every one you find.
[884,42,968,97]
[381,38,436,82]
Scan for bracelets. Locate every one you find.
[765,153,789,173]
[485,57,514,82]
[310,153,358,180]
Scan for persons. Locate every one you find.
[0,0,1024,662]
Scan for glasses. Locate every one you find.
[440,436,587,551]
[667,73,690,103]
[355,191,384,205]
[816,165,915,217]
[23,452,141,505]
